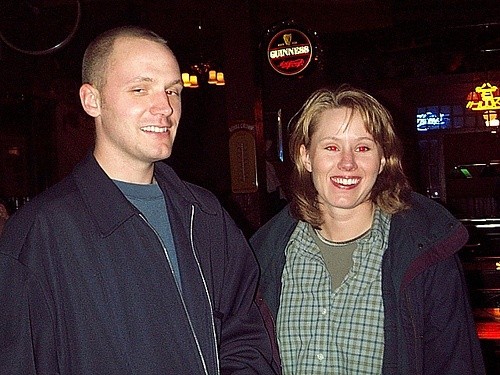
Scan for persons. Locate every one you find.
[0,14,282,375]
[229,83,488,375]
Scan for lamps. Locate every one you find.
[179,57,225,88]
